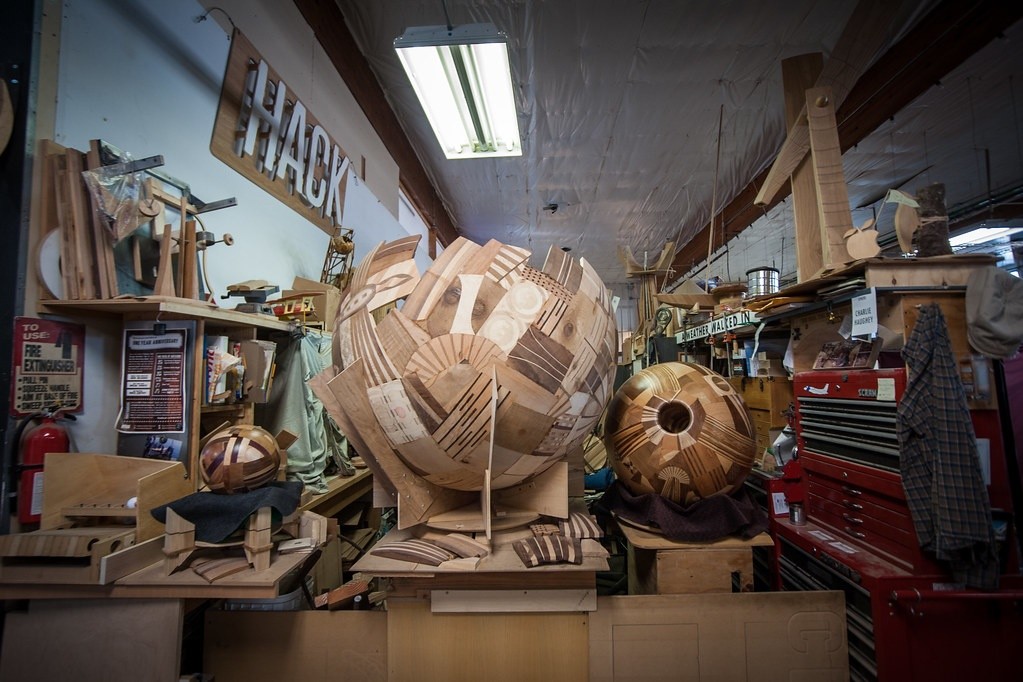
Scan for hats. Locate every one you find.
[964,265,1023,359]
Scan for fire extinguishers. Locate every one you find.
[16,405,70,531]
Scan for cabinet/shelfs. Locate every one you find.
[38,295,372,519]
[674,252,1006,471]
[611,509,774,596]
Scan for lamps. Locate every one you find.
[392,0,523,160]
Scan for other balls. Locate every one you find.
[602,359,758,511]
[198,422,282,494]
